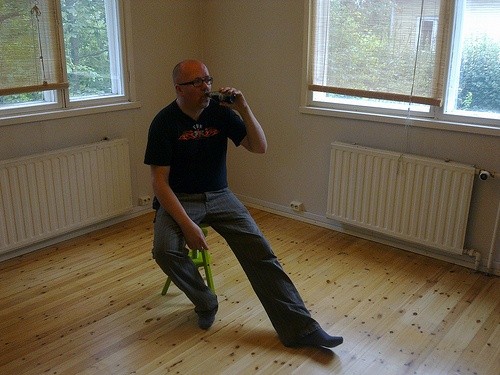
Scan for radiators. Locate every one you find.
[0,137,134,255]
[326,141,476,256]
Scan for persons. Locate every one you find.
[142,58,343,348]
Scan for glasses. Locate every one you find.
[178,77,213,88]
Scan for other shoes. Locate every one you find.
[290,327,343,347]
[199,298,218,329]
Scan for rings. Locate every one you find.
[199,246,204,252]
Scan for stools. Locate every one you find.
[161,221,216,296]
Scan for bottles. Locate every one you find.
[205,91,237,104]
